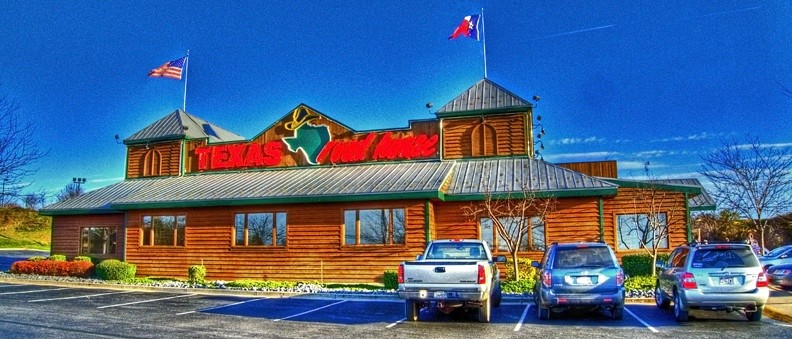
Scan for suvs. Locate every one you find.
[654,242,771,323]
[531,242,628,321]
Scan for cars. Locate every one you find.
[758,244,792,276]
[767,264,792,292]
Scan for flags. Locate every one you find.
[448,15,480,41]
[146,57,186,80]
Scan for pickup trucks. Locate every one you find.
[397,240,508,323]
